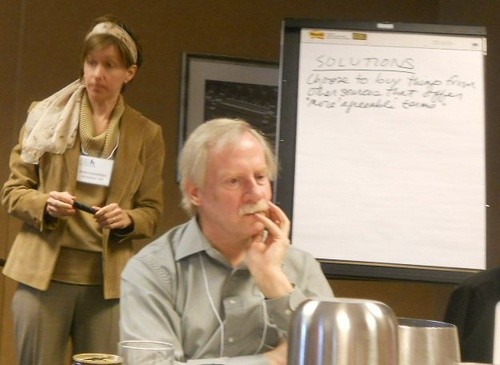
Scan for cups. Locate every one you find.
[117,339,175,365]
[397,317,460,365]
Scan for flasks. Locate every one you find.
[286,297,397,365]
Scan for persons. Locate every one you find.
[1,14,166,365]
[117,118,338,365]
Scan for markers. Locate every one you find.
[72,200,97,214]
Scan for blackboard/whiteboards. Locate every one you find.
[272,13,500,289]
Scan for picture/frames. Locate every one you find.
[178,51,281,189]
